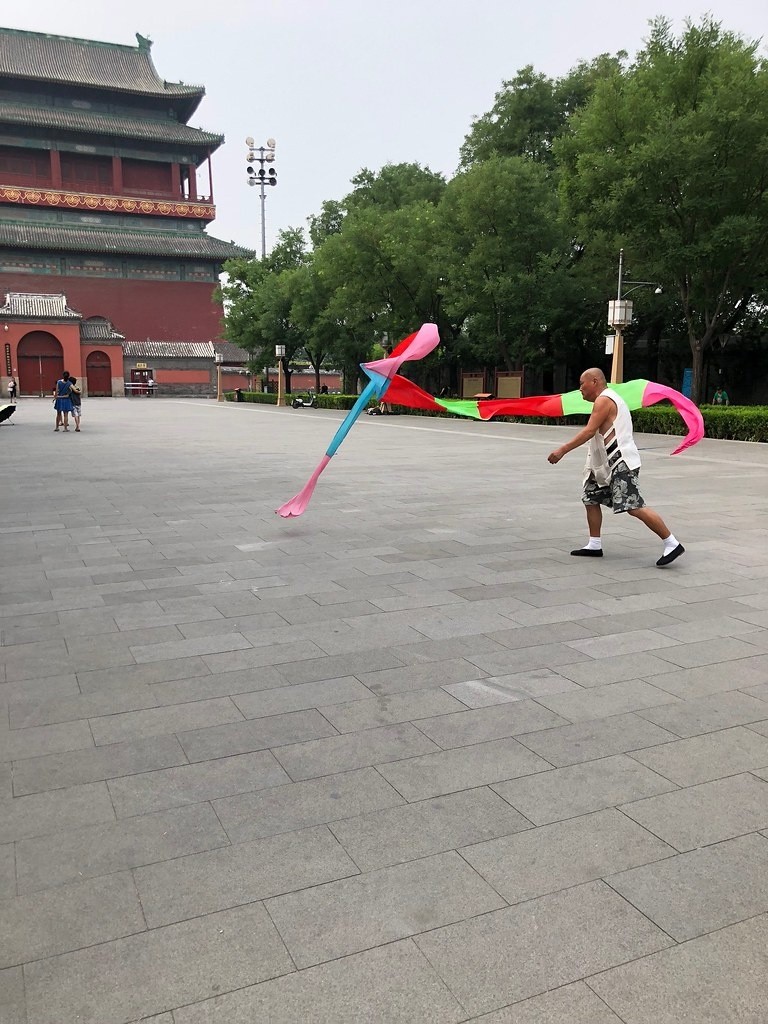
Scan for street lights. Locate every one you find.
[246,134,278,261]
[612,278,665,301]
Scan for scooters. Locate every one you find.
[291,388,319,409]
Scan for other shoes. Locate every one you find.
[55,429,59,431]
[59,423,63,426]
[63,423,69,426]
[64,430,70,432]
[656,543,685,565]
[75,429,80,431]
[571,549,604,557]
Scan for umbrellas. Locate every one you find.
[0,404,16,425]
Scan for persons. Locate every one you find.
[320,383,328,394]
[148,377,154,395]
[8,377,17,403]
[712,386,729,406]
[548,368,685,566]
[440,385,448,398]
[53,371,81,432]
[366,403,376,411]
